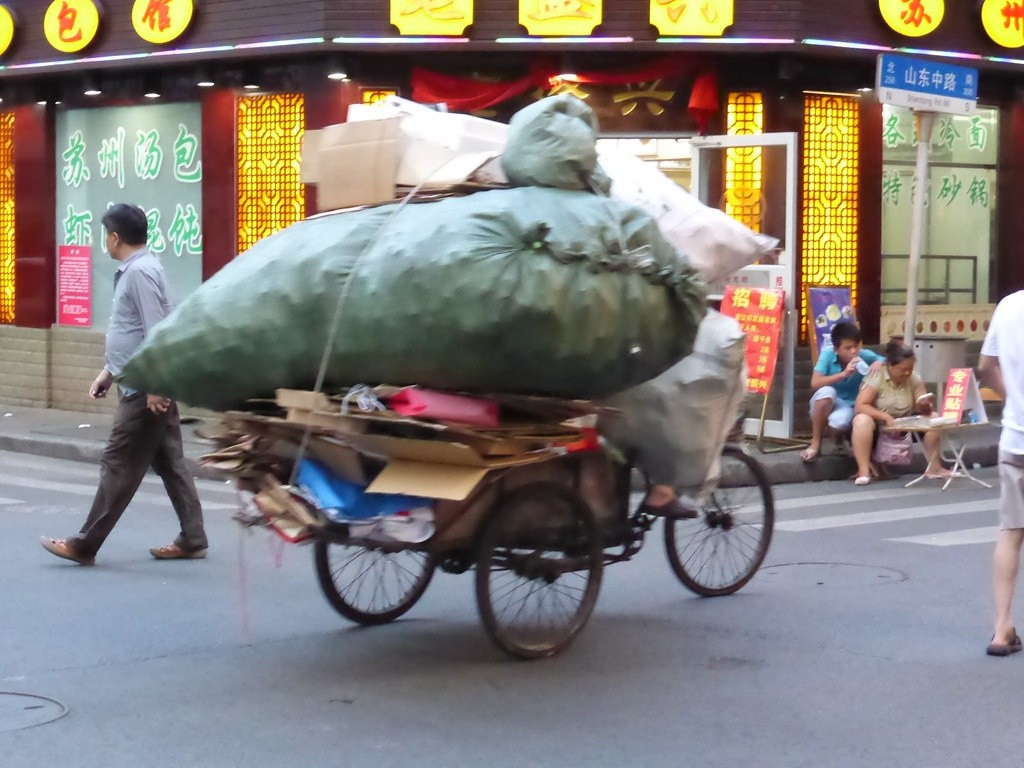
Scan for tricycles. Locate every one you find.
[234,408,776,664]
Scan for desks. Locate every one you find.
[879,417,993,492]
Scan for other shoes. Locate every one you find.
[855,476,870,485]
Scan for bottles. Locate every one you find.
[855,356,870,375]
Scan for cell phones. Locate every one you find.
[916,393,933,404]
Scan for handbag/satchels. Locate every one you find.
[872,431,913,465]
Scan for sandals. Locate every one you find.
[149,543,208,559]
[40,535,96,565]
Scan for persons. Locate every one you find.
[851,343,952,485]
[41,204,209,564]
[800,323,886,463]
[643,485,699,525]
[974,290,1024,654]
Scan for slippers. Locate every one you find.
[643,497,698,518]
[833,444,848,456]
[800,452,821,463]
[987,626,1023,654]
